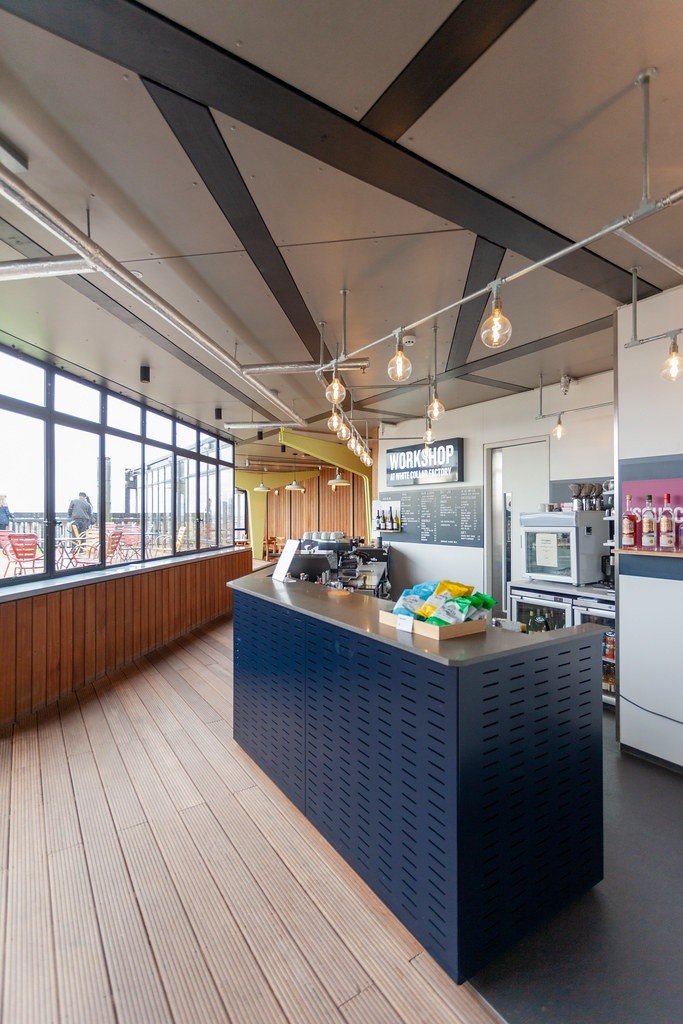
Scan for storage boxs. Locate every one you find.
[378,609,486,640]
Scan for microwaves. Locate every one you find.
[518,510,610,587]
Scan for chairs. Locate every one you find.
[0,522,187,578]
[262,536,285,561]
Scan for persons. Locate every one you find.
[0,496,14,549]
[67,492,94,554]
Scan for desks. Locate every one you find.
[121,532,158,561]
[37,537,86,569]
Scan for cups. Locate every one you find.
[287,573,309,582]
[539,478,614,516]
[303,531,344,540]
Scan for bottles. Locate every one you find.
[376,510,380,529]
[387,506,393,530]
[678,521,683,553]
[393,510,399,530]
[380,510,386,530]
[581,614,616,698]
[659,494,675,552]
[386,510,389,530]
[641,495,657,551]
[528,607,566,635]
[622,495,639,550]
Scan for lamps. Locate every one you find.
[327,468,351,485]
[284,456,303,490]
[253,467,271,491]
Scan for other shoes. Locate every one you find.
[79,549,86,554]
[68,550,75,554]
